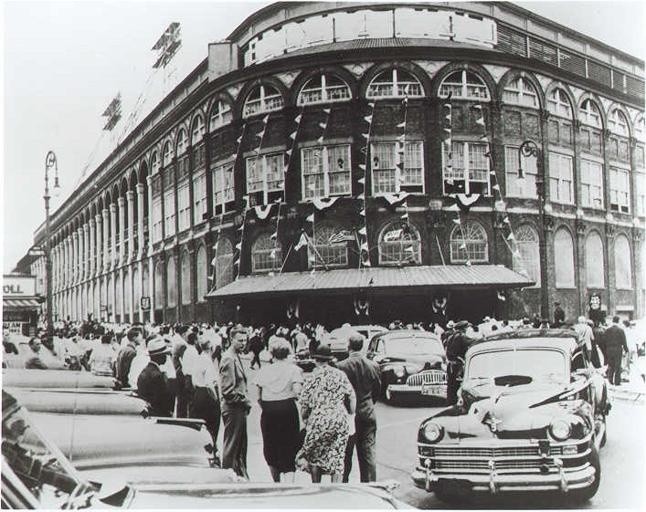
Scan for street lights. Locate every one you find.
[43,148,62,338]
[517,140,552,327]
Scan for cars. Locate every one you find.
[295,323,388,372]
[415,331,613,506]
[369,329,451,405]
[2,328,423,510]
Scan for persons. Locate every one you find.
[392,320,445,337]
[482,315,548,339]
[254,339,305,484]
[120,319,234,416]
[294,344,357,483]
[441,320,474,403]
[551,301,635,386]
[334,335,381,482]
[249,320,317,369]
[216,327,251,479]
[4,316,125,377]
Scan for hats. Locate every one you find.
[447,320,468,330]
[144,338,171,357]
[311,343,334,360]
